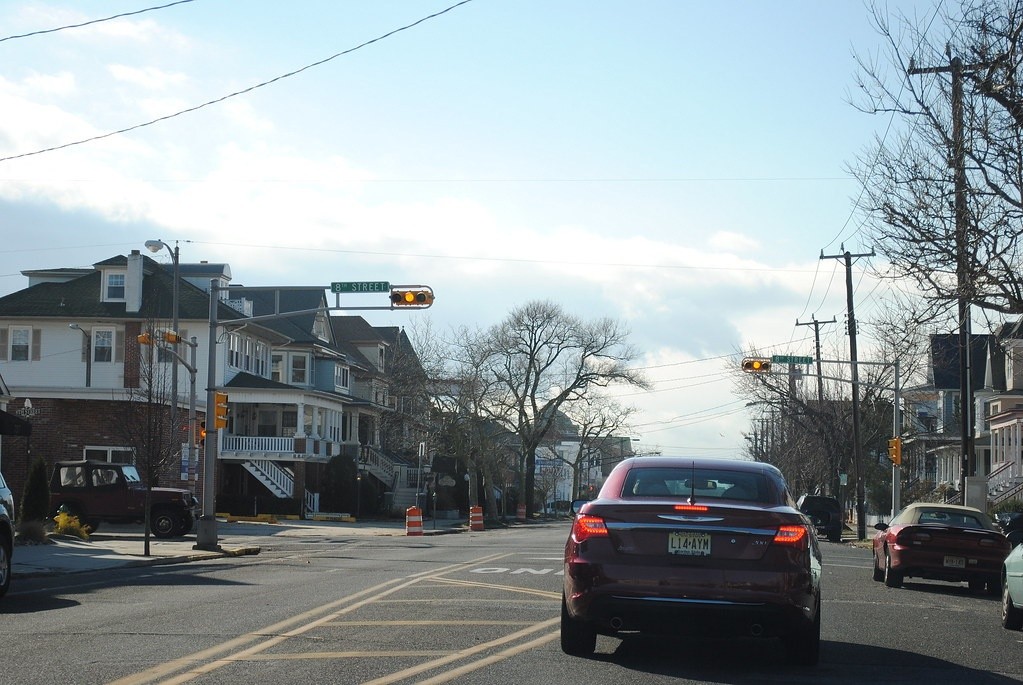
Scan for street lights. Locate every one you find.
[145,240,178,487]
[69,324,92,387]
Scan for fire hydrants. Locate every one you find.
[53,503,70,531]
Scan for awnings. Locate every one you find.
[0,409,32,436]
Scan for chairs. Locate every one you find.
[634,478,670,495]
[720,484,754,500]
[104,471,113,484]
[947,516,964,522]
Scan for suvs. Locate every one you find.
[47,459,197,539]
[0,472,14,591]
[796,493,842,543]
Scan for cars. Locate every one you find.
[1000,529,1023,629]
[562,455,822,672]
[872,502,1012,596]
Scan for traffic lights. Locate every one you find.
[745,361,771,369]
[198,420,205,445]
[391,291,432,305]
[888,438,900,465]
[215,391,228,428]
